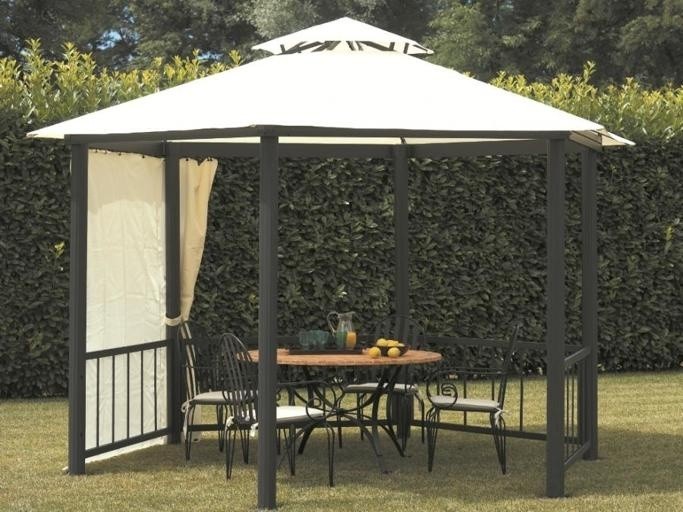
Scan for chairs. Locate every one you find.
[342,315,430,453]
[177,320,257,465]
[426,325,519,474]
[216,332,339,487]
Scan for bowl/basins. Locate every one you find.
[369,342,410,357]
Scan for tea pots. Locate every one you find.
[326,310,360,352]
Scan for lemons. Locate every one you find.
[368,338,404,358]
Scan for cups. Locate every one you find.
[298,329,330,351]
